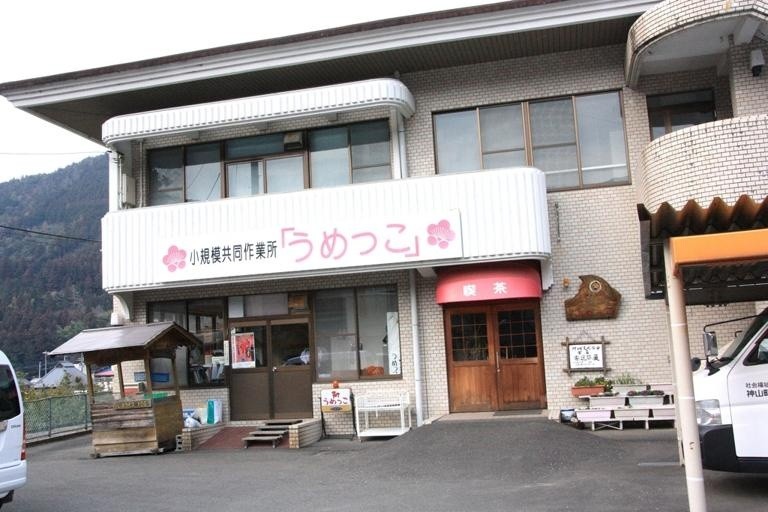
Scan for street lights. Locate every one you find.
[64,355,68,361]
[42,351,50,374]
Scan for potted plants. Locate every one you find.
[571,370,675,421]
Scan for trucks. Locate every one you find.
[676,303,767,477]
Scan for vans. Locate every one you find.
[0,350,31,509]
